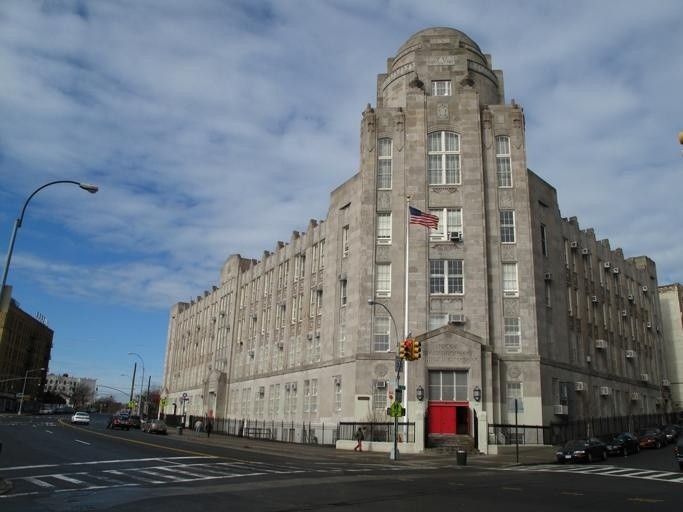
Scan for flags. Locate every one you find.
[408,205,439,231]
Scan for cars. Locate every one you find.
[38,406,97,414]
[556,437,607,463]
[107,413,139,430]
[606,432,640,456]
[71,411,89,425]
[639,424,683,463]
[141,419,166,434]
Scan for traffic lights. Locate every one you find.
[399,336,421,360]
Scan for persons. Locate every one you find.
[205,420,212,437]
[193,419,203,434]
[353,427,363,452]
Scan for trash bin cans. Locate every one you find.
[176,426,182,435]
[456,447,467,466]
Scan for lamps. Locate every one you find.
[416,385,424,401]
[474,385,480,400]
[587,354,591,363]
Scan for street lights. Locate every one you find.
[121,352,144,416]
[18,368,45,415]
[368,300,400,460]
[0,181,98,308]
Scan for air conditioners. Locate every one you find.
[663,378,670,387]
[257,386,264,394]
[276,340,283,349]
[656,397,662,406]
[639,373,649,382]
[450,230,461,241]
[306,331,319,340]
[545,272,553,281]
[247,350,254,358]
[574,382,585,392]
[448,313,464,324]
[337,273,346,280]
[570,241,662,332]
[627,349,634,359]
[596,340,607,350]
[631,392,638,401]
[283,382,296,391]
[334,377,340,386]
[375,380,386,389]
[599,387,609,397]
